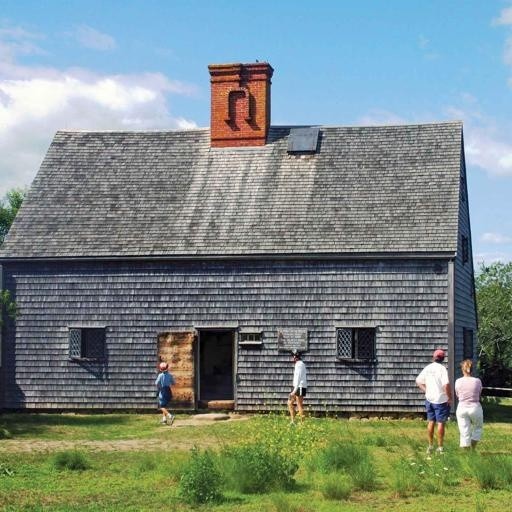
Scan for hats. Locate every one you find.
[160,362,168,372]
[434,350,445,357]
[291,349,302,357]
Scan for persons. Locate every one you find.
[154,361,176,426]
[452,359,484,453]
[286,347,308,427]
[414,348,453,454]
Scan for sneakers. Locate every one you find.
[286,421,296,427]
[427,447,444,455]
[160,415,175,425]
